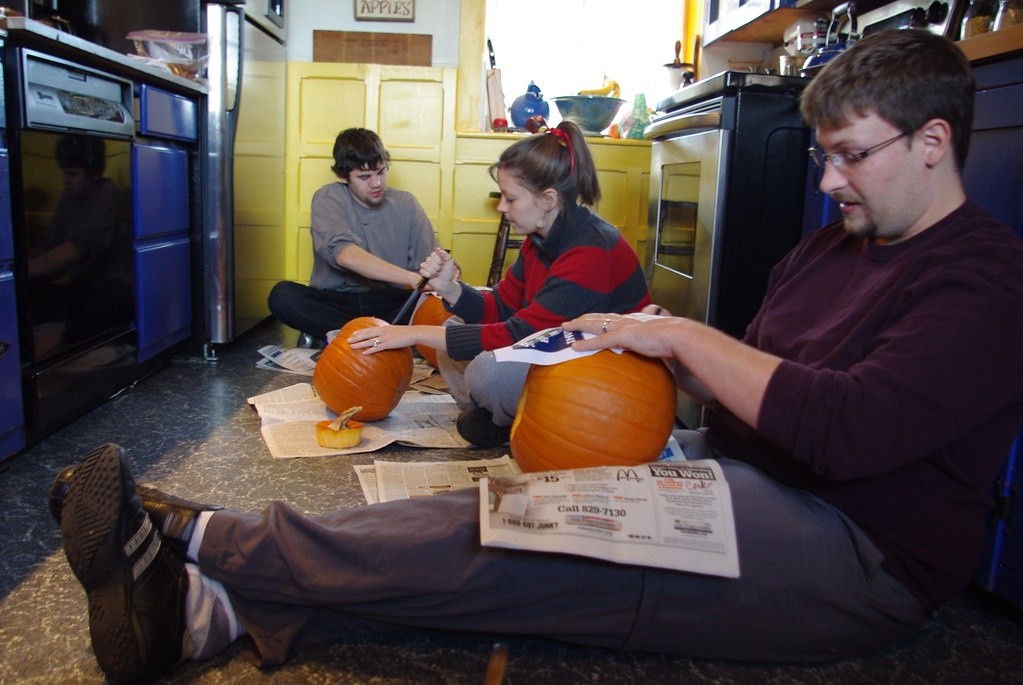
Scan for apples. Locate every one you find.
[610,124,621,138]
[527,114,546,134]
[493,118,507,128]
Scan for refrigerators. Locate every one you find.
[136,0,288,359]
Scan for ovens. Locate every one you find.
[637,97,828,340]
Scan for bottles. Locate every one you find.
[960,0,997,42]
[989,0,1023,32]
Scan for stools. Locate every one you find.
[487,191,523,288]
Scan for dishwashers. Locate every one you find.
[5,45,137,444]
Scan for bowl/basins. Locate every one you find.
[549,95,627,136]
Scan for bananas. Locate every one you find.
[577,79,621,98]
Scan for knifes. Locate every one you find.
[391,249,450,325]
[488,38,496,68]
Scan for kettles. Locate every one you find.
[800,2,861,78]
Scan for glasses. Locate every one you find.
[808,127,919,173]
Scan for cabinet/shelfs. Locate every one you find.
[702,0,817,49]
[131,86,197,382]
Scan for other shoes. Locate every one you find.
[297,332,321,349]
[457,407,513,449]
[323,329,341,349]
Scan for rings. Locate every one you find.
[373,337,379,347]
[444,257,452,261]
[602,319,611,334]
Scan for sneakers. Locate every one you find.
[50,465,224,564]
[61,444,189,685]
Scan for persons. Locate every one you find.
[347,119,654,447]
[268,127,462,349]
[48,29,1023,685]
[469,471,487,478]
